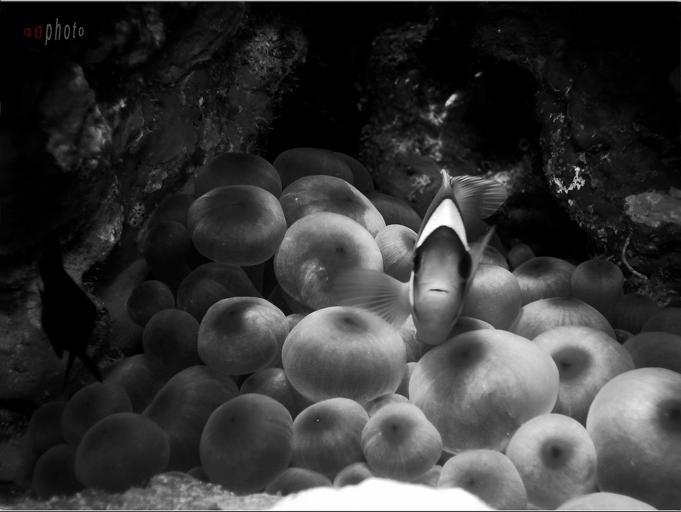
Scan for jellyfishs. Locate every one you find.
[36,145,681,512]
